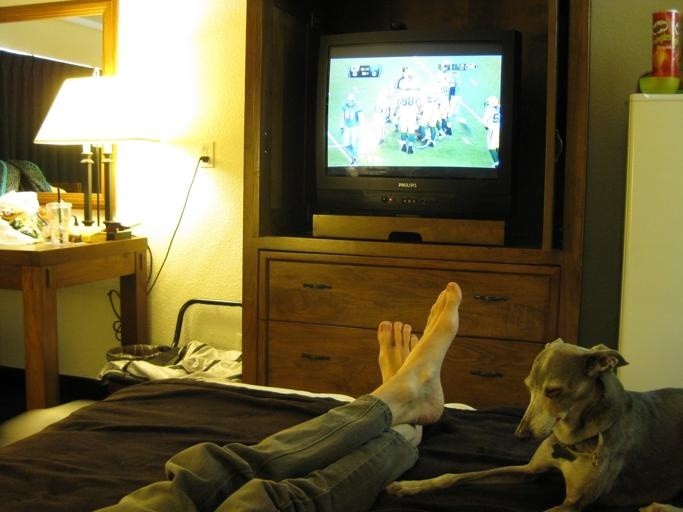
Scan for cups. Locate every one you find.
[46,201,72,245]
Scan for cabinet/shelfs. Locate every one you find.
[244,238,581,408]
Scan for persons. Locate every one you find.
[331,57,477,165]
[483,95,500,165]
[96,282,463,511]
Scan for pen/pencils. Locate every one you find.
[58,186,63,244]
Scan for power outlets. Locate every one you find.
[200,141,214,168]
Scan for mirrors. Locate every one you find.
[0,2,119,210]
[34,70,162,239]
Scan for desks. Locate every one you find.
[1,230,149,412]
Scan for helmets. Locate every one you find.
[485,95,498,107]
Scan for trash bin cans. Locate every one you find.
[107,344,170,361]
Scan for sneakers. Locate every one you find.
[400,144,414,154]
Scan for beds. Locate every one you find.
[3,379,683,512]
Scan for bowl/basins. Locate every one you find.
[640,76,680,94]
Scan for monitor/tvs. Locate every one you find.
[310,25,524,222]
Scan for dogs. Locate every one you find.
[385,338,683,512]
